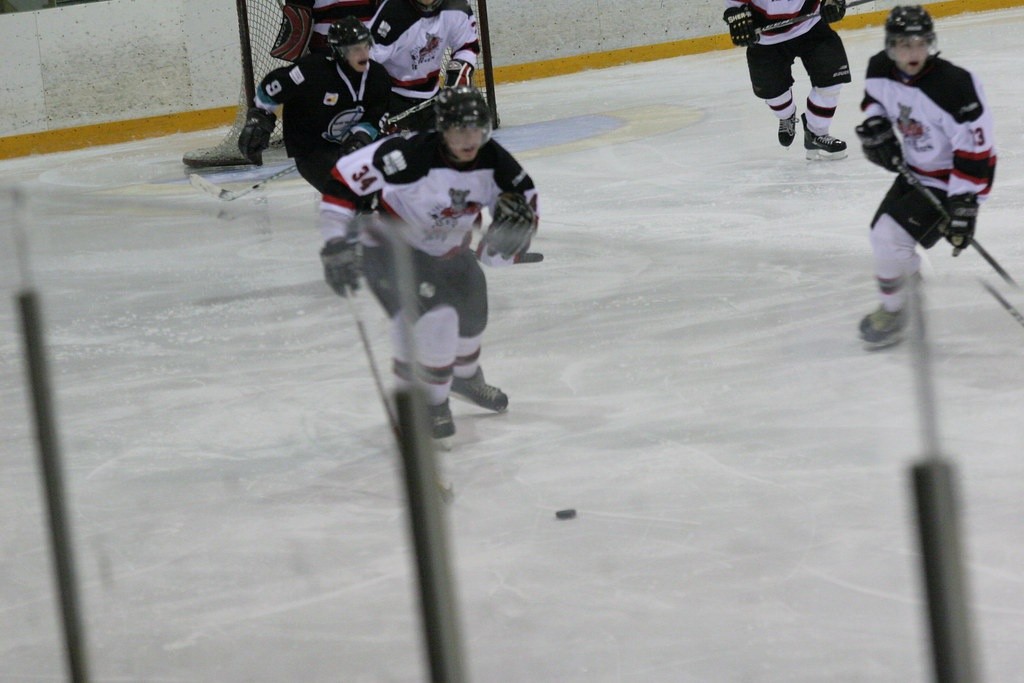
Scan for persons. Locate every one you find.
[237,0,480,204]
[722,0,852,160]
[318,84,539,451]
[854,5,998,351]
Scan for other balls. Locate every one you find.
[556,509,576,519]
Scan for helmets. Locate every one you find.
[884,5,939,61]
[328,15,370,47]
[434,85,491,126]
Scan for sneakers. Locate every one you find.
[451,368,508,412]
[804,132,848,161]
[431,411,458,442]
[778,113,800,147]
[859,303,913,345]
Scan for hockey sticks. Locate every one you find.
[190,165,294,201]
[755,0,874,34]
[893,156,1017,284]
[345,285,457,506]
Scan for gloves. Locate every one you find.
[323,243,360,298]
[237,109,276,165]
[856,121,904,174]
[821,0,847,21]
[723,5,756,47]
[945,203,979,257]
